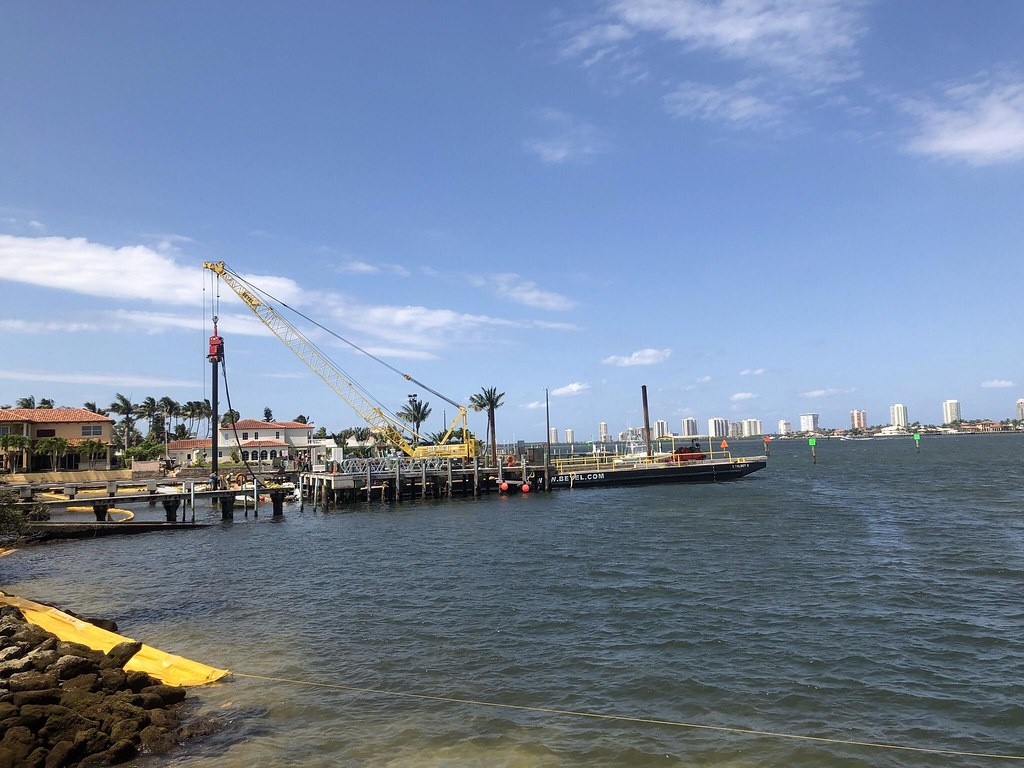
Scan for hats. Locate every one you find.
[298,452,301,455]
[304,454,307,456]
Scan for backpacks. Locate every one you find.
[209,473,213,478]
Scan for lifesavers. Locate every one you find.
[235,473,247,485]
[507,455,515,466]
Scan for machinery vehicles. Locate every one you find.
[201,261,475,462]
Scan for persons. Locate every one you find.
[507,450,515,467]
[209,469,218,491]
[301,454,309,472]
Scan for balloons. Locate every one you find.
[501,482,508,490]
[522,485,529,492]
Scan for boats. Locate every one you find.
[539,385,767,488]
